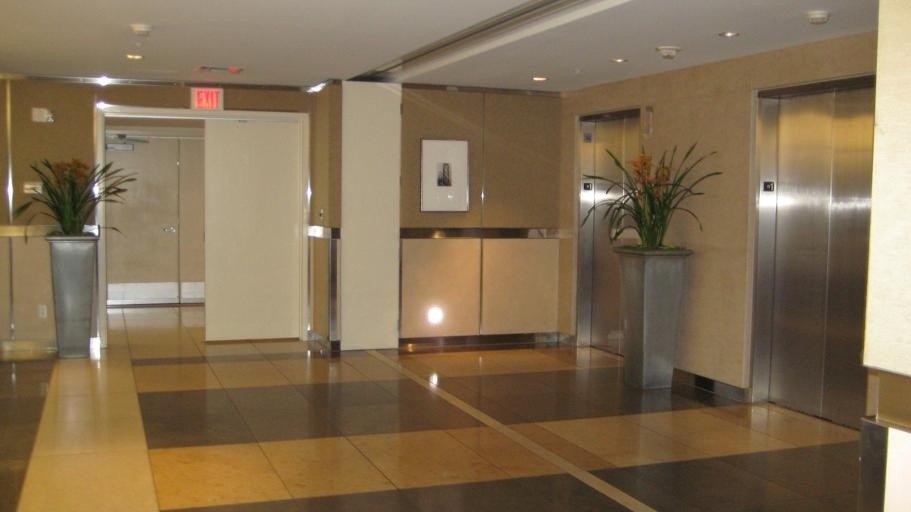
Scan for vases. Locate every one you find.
[611,246,695,391]
[45,225,100,358]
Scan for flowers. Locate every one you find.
[579,141,723,245]
[12,154,139,242]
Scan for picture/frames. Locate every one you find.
[420,135,472,211]
